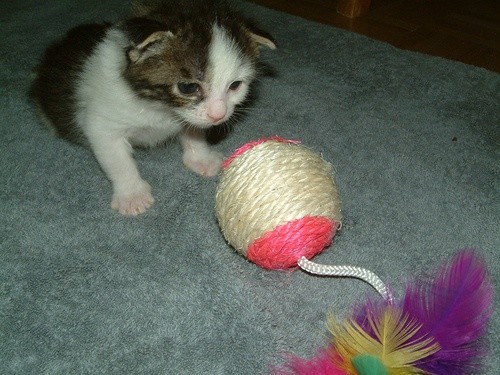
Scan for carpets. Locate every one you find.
[1,0,500,374]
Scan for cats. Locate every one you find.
[29,2,277,219]
